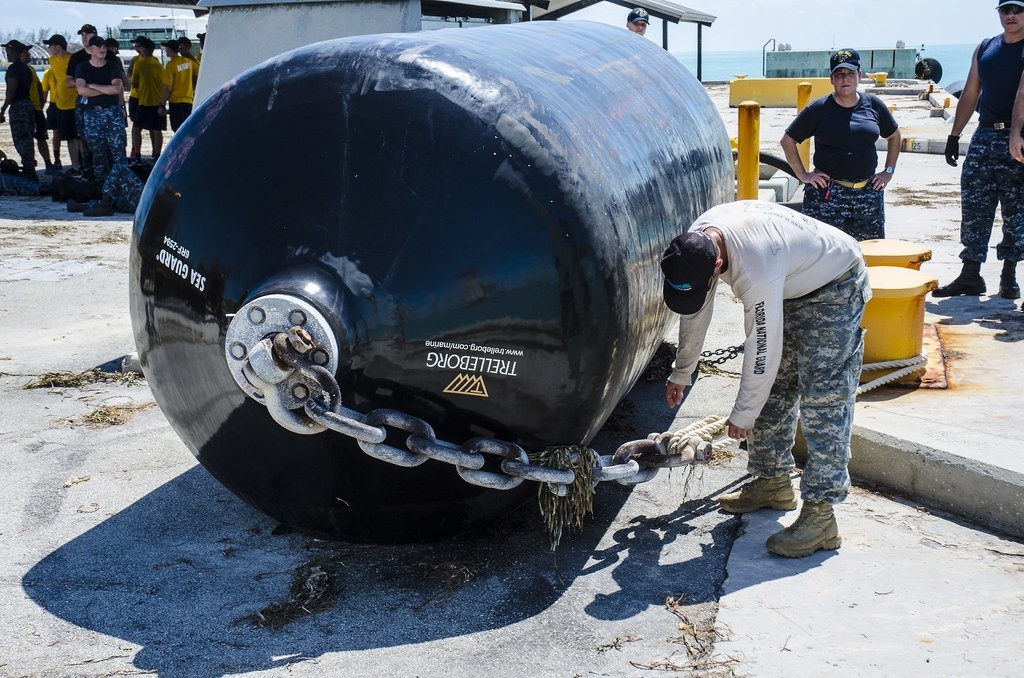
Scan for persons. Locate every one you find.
[0,24,207,216]
[1009,71,1024,164]
[660,199,873,559]
[931,0,1024,298]
[780,48,900,244]
[626,8,650,36]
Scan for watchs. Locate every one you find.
[885,166,894,174]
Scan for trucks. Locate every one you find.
[107,15,189,90]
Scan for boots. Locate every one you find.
[764,500,842,558]
[82,195,114,216]
[66,198,88,212]
[931,258,987,297]
[717,473,798,513]
[998,259,1020,298]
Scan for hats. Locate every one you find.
[994,0,1024,10]
[659,228,717,317]
[1,40,33,53]
[88,36,107,47]
[78,24,97,34]
[627,8,650,25]
[829,47,861,77]
[42,34,67,50]
[106,38,120,54]
[197,33,205,41]
[129,35,192,51]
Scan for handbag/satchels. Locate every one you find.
[49,173,86,203]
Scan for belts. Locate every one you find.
[813,168,876,190]
[979,121,1010,130]
[824,259,866,285]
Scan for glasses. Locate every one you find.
[998,5,1024,15]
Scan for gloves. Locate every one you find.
[944,135,960,167]
